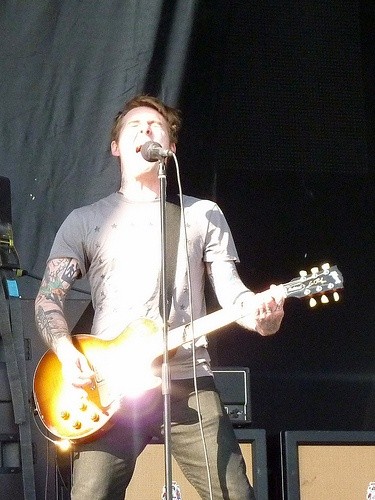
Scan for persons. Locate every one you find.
[33,95,286,500]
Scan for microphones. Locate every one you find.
[140,140,174,163]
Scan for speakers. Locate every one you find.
[208,365,253,430]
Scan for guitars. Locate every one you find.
[32,260,345,447]
